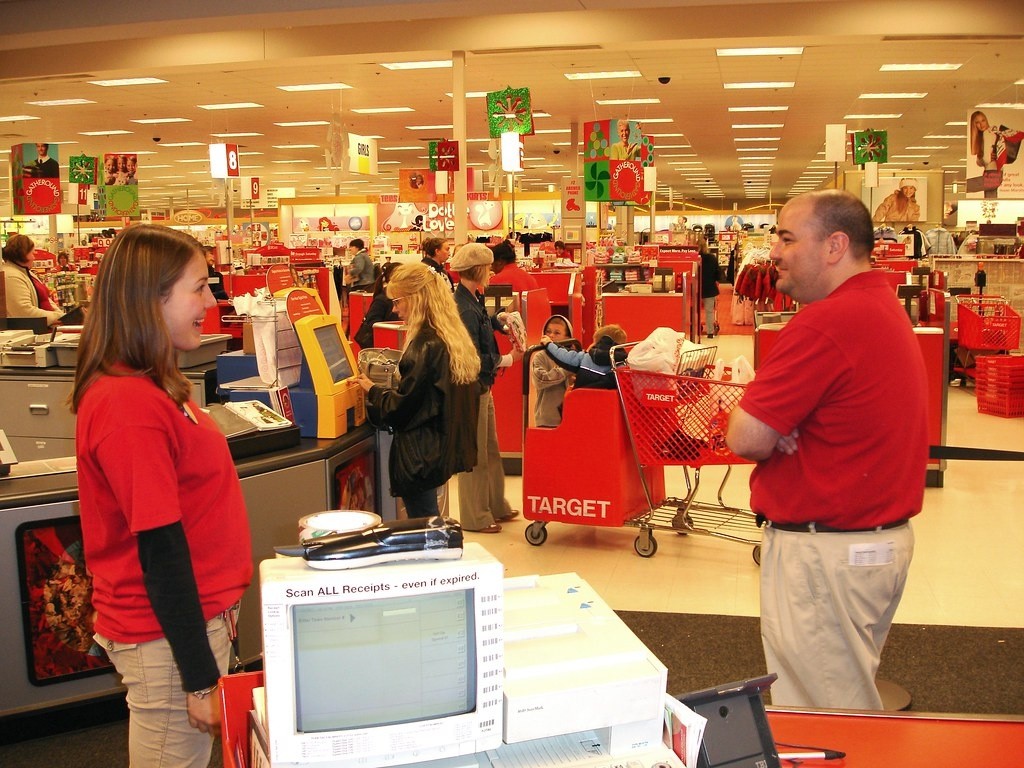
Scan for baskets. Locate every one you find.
[975,353,1024,419]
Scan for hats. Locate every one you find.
[449,243,495,272]
[898,177,919,190]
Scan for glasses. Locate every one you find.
[390,296,406,305]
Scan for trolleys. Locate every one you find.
[698,281,721,335]
[953,294,1021,388]
[521,337,764,568]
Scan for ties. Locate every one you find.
[37,156,45,170]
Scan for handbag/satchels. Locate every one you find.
[991,124,1024,163]
[357,345,404,390]
[711,355,755,414]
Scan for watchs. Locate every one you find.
[193,683,218,700]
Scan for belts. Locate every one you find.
[755,513,908,535]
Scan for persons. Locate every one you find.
[31,143,59,178]
[966,112,1008,199]
[696,238,722,339]
[449,244,519,533]
[349,231,573,357]
[104,155,138,185]
[873,179,919,224]
[203,246,235,312]
[67,224,253,768]
[675,217,688,231]
[727,190,928,710]
[529,315,629,428]
[410,175,424,190]
[0,233,78,327]
[609,120,641,160]
[348,261,482,518]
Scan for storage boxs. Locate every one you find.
[0,333,58,367]
[50,338,78,367]
[0,330,34,353]
[176,333,233,368]
[242,318,256,354]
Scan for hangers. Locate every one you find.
[906,224,913,230]
[935,224,942,230]
[878,223,886,230]
[746,242,776,267]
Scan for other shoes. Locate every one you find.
[466,523,503,534]
[493,509,520,523]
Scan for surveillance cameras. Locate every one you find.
[316,187,319,190]
[234,190,236,192]
[553,151,560,154]
[923,162,928,165]
[706,180,709,182]
[747,181,751,183]
[152,138,161,142]
[692,198,694,200]
[658,77,670,84]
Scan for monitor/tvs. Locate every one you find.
[294,315,360,395]
[259,541,502,768]
